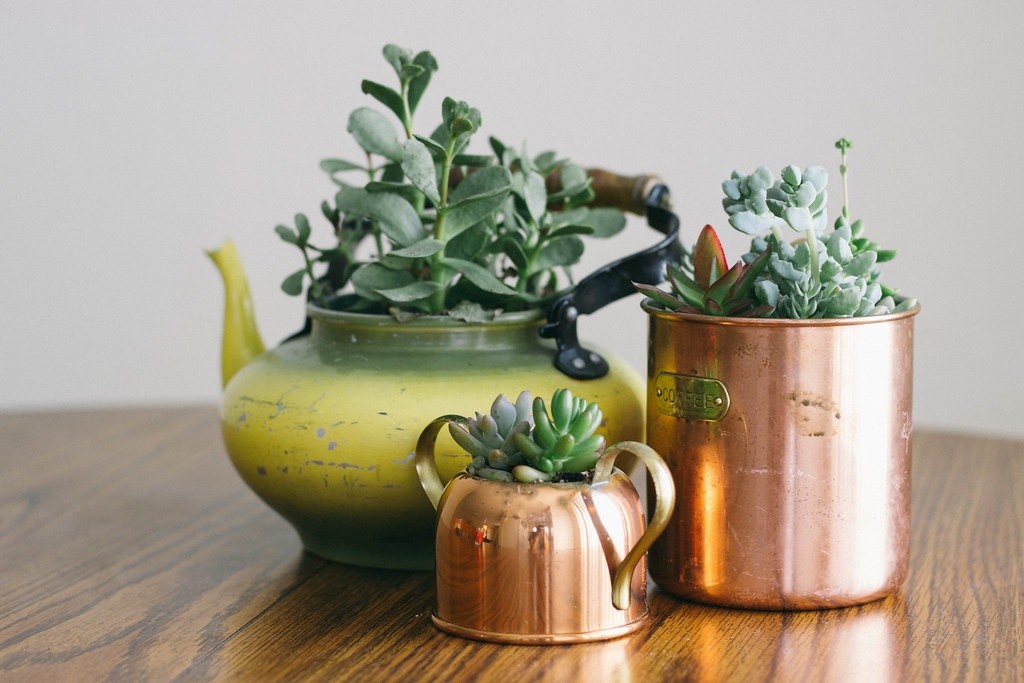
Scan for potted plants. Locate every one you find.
[631,136,921,611]
[204,44,685,574]
[413,387,676,647]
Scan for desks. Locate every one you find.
[1,409,1022,682]
